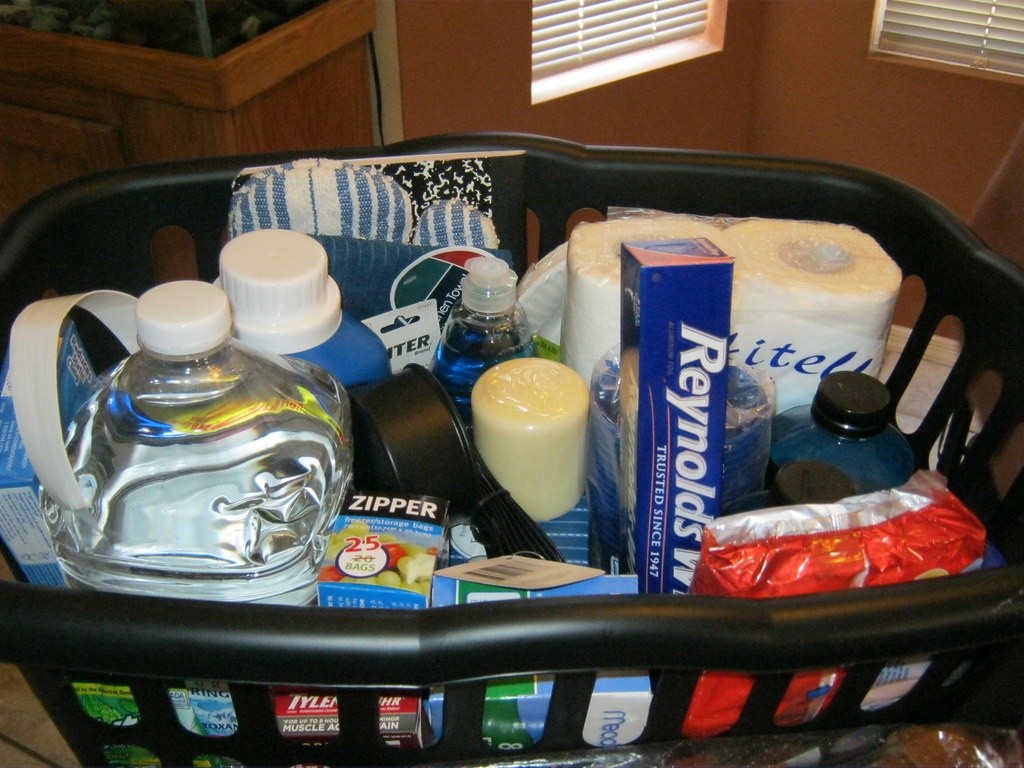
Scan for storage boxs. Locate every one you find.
[0,123,1024,768]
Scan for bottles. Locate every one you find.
[5,279,354,607]
[208,228,392,393]
[587,344,771,572]
[432,256,535,442]
[766,369,914,505]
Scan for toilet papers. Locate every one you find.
[566,208,902,421]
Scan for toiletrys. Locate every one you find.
[617,233,736,606]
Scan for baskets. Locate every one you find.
[0,128,1023,768]
